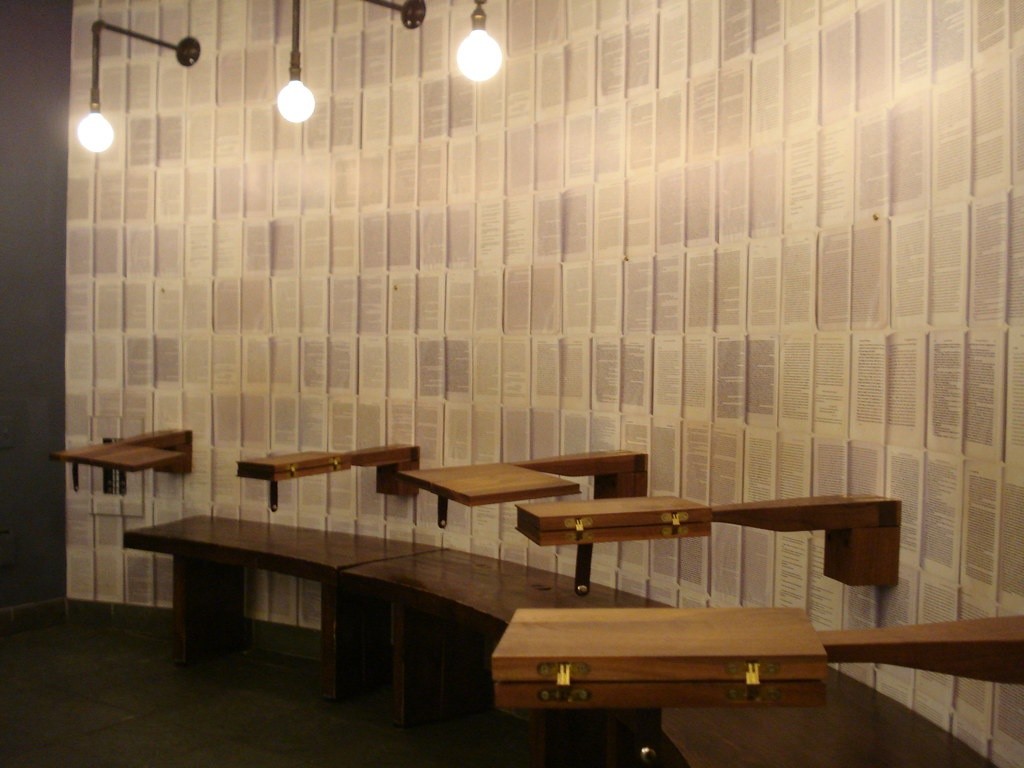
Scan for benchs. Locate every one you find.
[605,665,998,767]
[121,513,449,699]
[342,548,673,768]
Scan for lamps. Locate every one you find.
[456,0,503,82]
[276,0,426,124]
[77,18,199,152]
